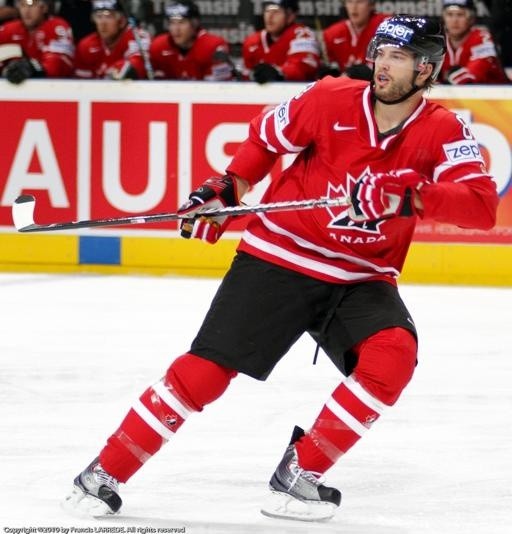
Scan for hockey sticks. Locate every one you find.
[12,194,350,232]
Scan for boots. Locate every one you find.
[269,425,342,510]
[72,455,123,514]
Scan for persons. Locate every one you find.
[72,0,157,82]
[145,0,234,82]
[73,12,501,515]
[433,0,512,85]
[321,0,395,81]
[235,0,323,85]
[0,1,76,84]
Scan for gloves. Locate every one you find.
[175,173,240,244]
[348,173,417,223]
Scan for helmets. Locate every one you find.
[366,13,449,83]
[16,0,62,15]
[164,1,203,32]
[442,1,478,24]
[261,0,301,17]
[90,0,132,22]
